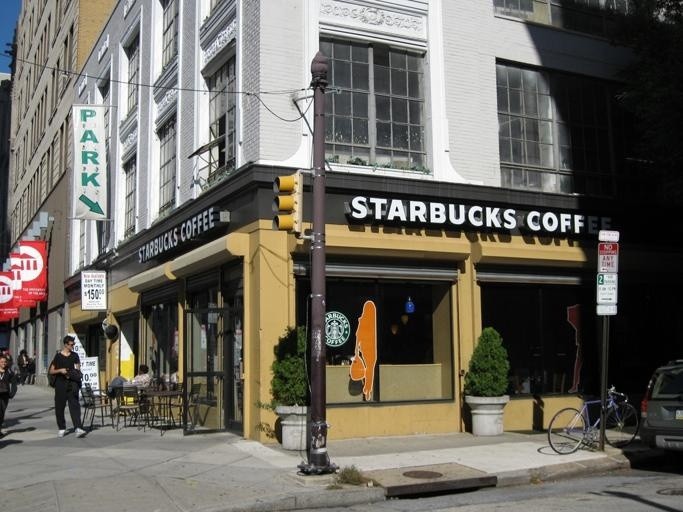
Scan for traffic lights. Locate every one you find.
[269,171,301,235]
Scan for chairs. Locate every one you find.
[80,376,201,436]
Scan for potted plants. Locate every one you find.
[251,324,311,451]
[463,327,511,436]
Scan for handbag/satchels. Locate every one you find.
[47,353,57,388]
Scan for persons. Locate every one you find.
[131,364,151,402]
[0,347,36,439]
[515,372,531,395]
[49,335,87,438]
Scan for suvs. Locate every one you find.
[638,358,683,459]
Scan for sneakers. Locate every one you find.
[58,429,66,438]
[75,429,87,438]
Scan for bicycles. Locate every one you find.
[544,382,641,456]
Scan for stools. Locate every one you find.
[538,393,587,430]
[503,395,538,432]
